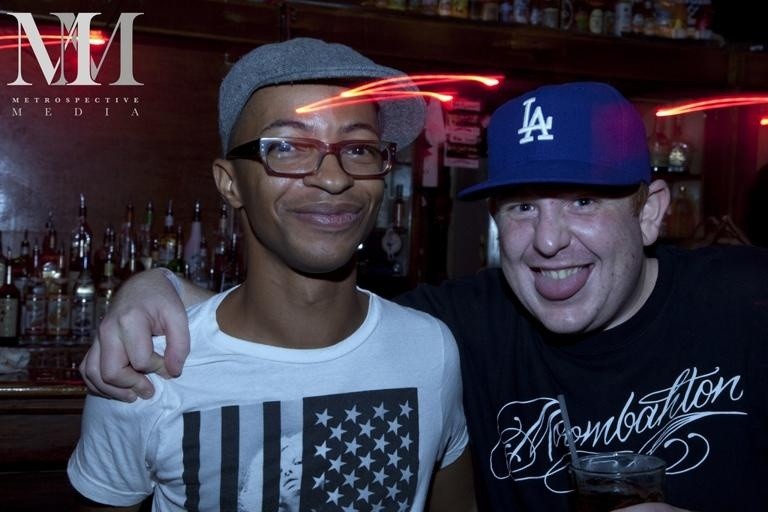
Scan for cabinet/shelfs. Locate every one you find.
[358,125,428,298]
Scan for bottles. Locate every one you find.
[369,1,715,39]
[388,184,411,274]
[1,194,246,346]
[644,111,696,236]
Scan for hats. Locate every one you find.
[455,82,651,202]
[218,37,426,159]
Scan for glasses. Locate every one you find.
[226,137,397,179]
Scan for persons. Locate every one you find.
[77,80,768,510]
[65,36,478,511]
